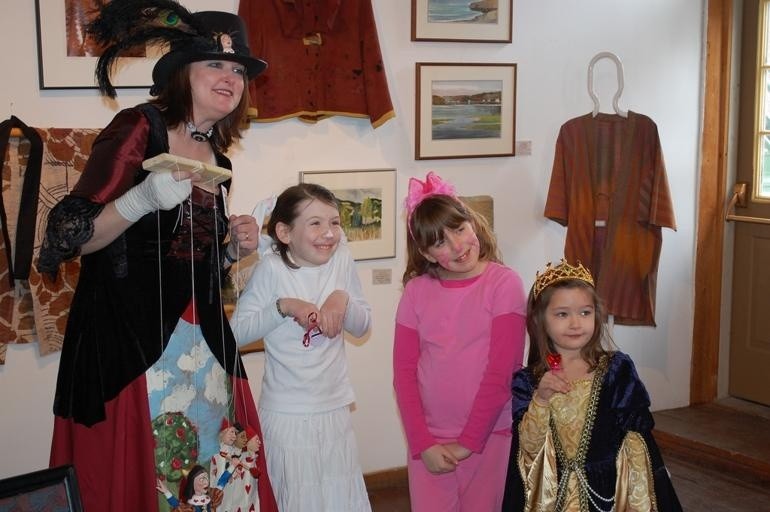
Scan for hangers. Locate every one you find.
[560,52,658,134]
[0,103,115,141]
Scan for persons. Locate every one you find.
[392,175,526,510]
[510,262,683,512]
[38,12,278,512]
[154,415,263,512]
[227,184,375,512]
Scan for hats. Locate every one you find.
[232,422,244,434]
[246,423,257,442]
[152,11,269,82]
[219,416,233,432]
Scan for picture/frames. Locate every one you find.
[35,0,171,89]
[416,62,518,160]
[411,0,513,43]
[301,169,396,261]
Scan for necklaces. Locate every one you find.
[184,118,214,141]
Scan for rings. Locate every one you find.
[246,231,249,240]
